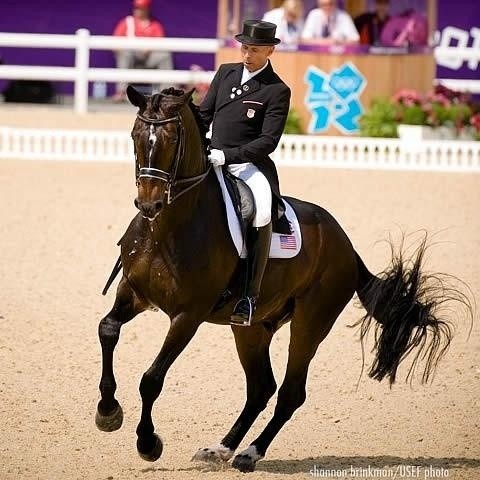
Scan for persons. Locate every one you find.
[196,19,292,328]
[112,1,175,103]
[223,1,430,51]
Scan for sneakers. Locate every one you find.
[112,91,127,102]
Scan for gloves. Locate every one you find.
[208,149,225,168]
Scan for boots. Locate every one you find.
[230,220,273,323]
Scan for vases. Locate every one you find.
[398,124,476,140]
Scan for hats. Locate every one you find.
[234,20,280,45]
[133,0,151,8]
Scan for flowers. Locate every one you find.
[370,89,480,135]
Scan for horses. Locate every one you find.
[88,87,475,475]
